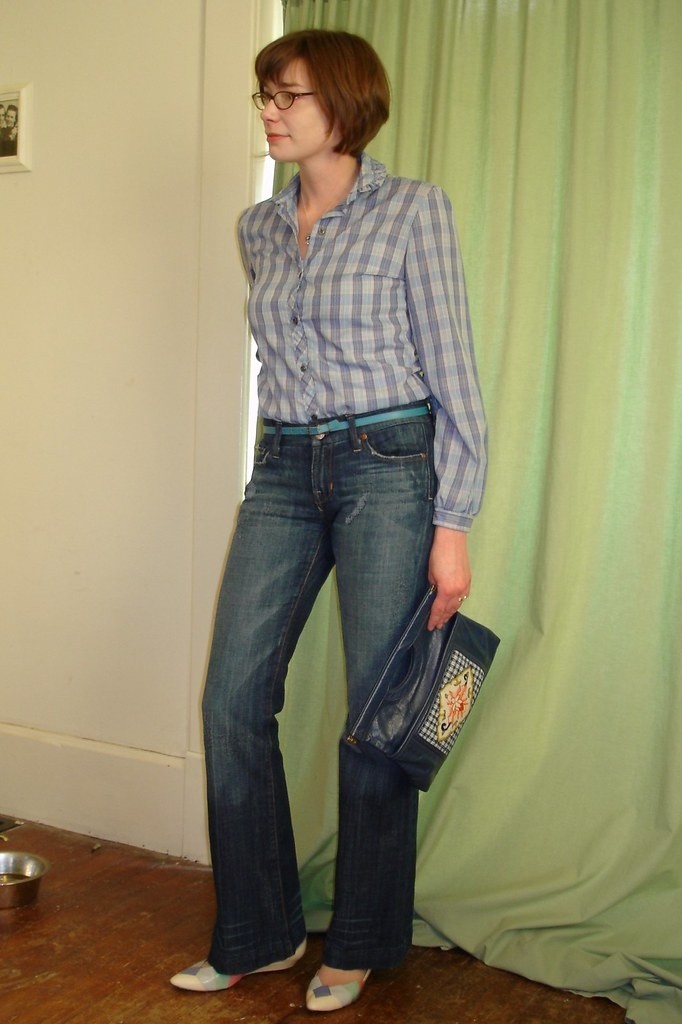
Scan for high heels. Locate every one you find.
[169,932,307,992]
[305,967,371,1011]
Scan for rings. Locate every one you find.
[458,595,468,602]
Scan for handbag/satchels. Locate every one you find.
[347,583,501,792]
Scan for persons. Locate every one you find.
[166,31,486,1011]
[0,105,18,156]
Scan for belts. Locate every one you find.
[262,405,428,435]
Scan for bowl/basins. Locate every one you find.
[0,851,51,909]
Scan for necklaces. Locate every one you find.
[300,192,342,245]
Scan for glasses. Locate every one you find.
[251,91,318,111]
